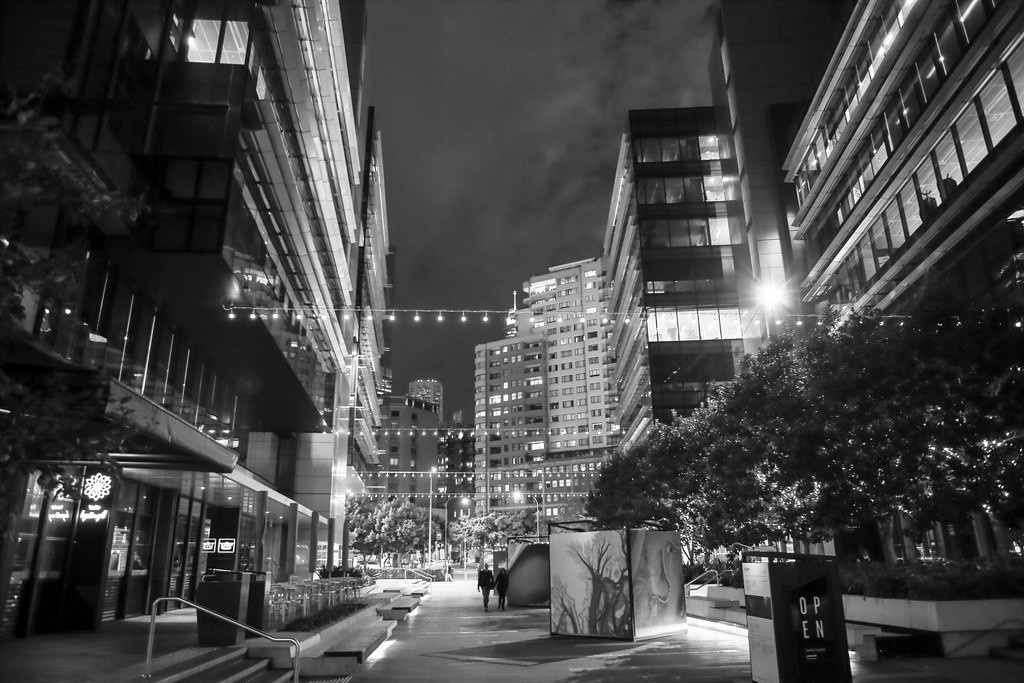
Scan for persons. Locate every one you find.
[476,561,494,611]
[494,568,508,610]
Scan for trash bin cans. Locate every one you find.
[195,570,272,647]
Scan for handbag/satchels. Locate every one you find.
[489,581,496,589]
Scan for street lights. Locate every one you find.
[445,496,470,559]
[428,466,438,564]
[513,491,541,544]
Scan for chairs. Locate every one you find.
[268,577,363,624]
